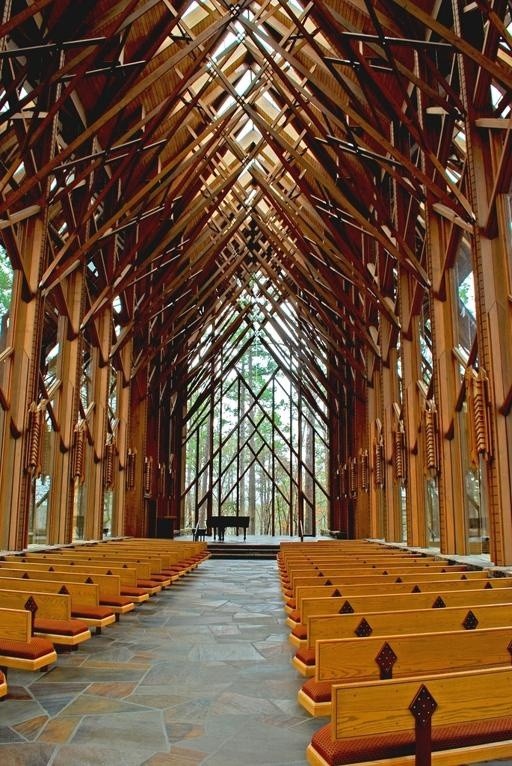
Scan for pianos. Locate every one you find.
[207,516,251,541]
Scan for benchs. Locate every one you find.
[0,537,212,697]
